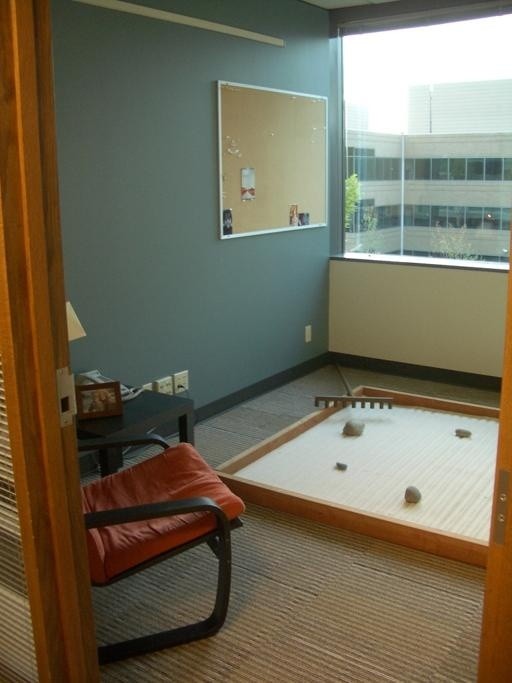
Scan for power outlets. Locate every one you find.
[174,368,189,394]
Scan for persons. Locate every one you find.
[88,391,114,413]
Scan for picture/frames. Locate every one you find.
[76,380,124,420]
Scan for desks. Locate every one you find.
[71,382,195,478]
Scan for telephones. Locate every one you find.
[76,370,134,402]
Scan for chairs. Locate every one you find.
[76,433,246,667]
